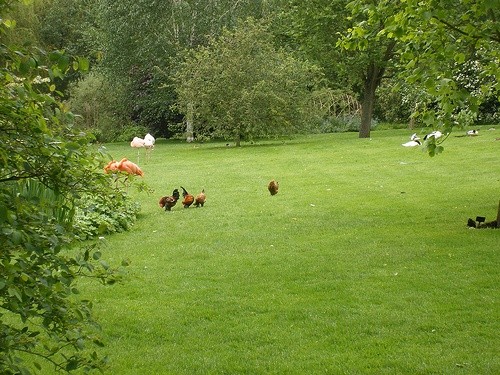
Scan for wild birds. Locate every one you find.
[143,133,155,154]
[180,186,194,208]
[130,136,144,155]
[193,189,206,207]
[104,158,144,192]
[159,189,180,211]
[467,130,478,135]
[268,180,279,196]
[401,130,441,147]
